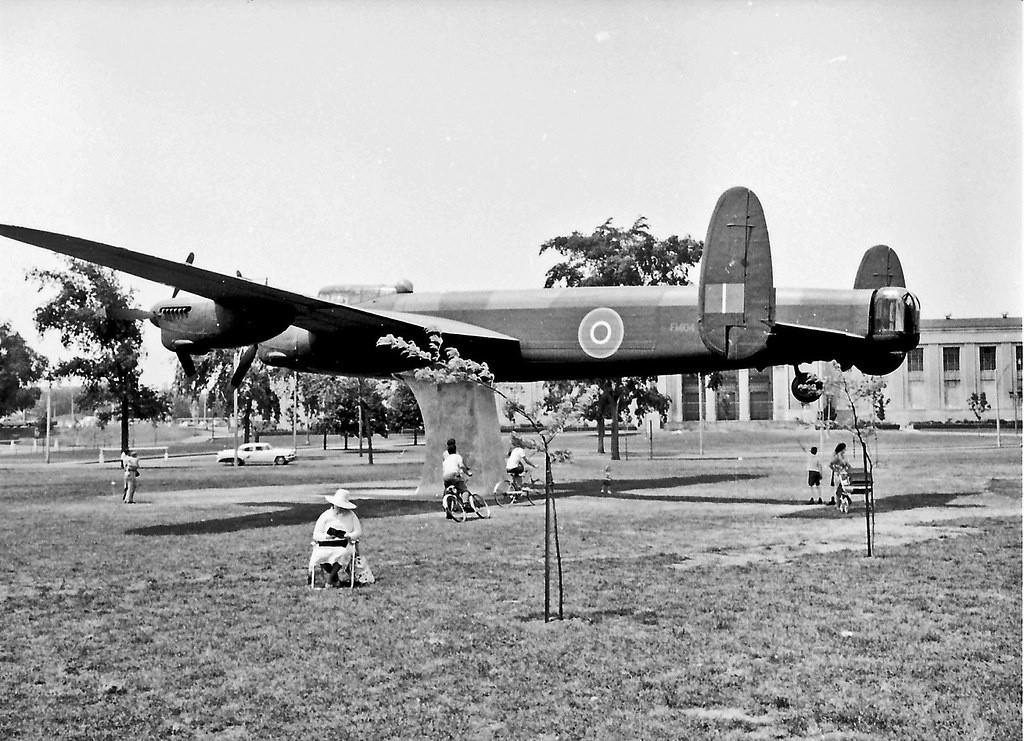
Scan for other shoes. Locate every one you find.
[128,500,136,503]
[809,498,815,504]
[325,582,334,589]
[335,580,341,587]
[463,503,475,513]
[818,498,823,504]
[446,512,453,519]
[122,493,126,501]
[521,487,532,491]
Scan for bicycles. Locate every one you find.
[835,471,852,513]
[442,475,491,523]
[493,465,547,508]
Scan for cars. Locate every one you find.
[217,442,297,467]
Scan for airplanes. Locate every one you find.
[0,184,921,402]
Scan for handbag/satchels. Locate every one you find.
[134,470,140,477]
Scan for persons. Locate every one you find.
[313,489,363,589]
[602,466,612,494]
[443,438,476,519]
[253,427,260,450]
[506,444,537,503]
[798,440,854,509]
[118,442,141,503]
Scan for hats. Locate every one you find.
[325,488,357,510]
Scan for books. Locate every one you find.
[327,528,346,538]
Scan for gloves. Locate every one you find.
[327,528,346,539]
